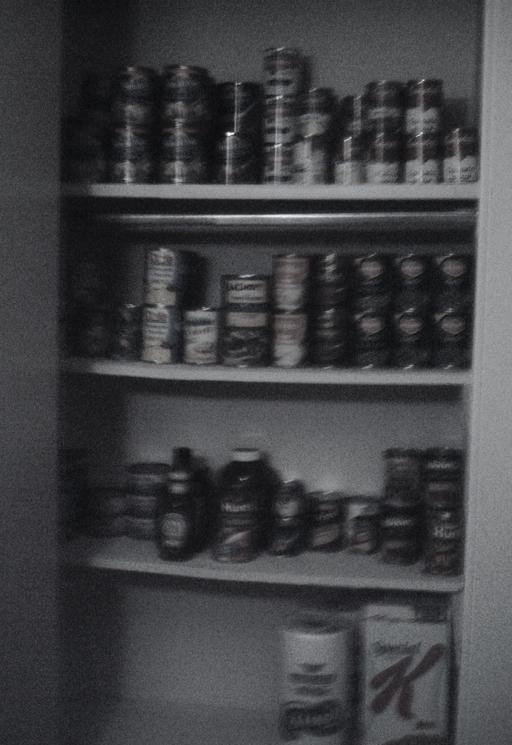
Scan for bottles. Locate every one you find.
[215,448,279,564]
[156,447,206,562]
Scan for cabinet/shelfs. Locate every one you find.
[51,179,480,592]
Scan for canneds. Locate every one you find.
[213,446,462,577]
[58,454,169,540]
[74,47,476,186]
[79,243,470,370]
